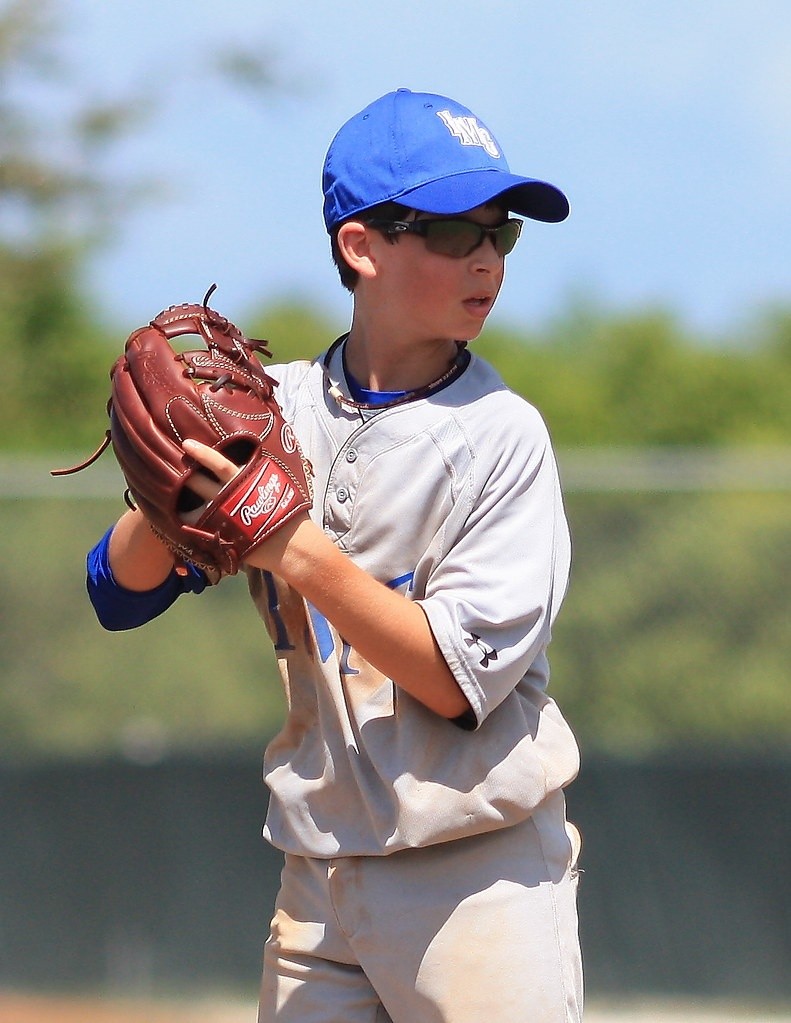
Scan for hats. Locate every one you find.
[323,88,570,234]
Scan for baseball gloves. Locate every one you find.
[106,302,315,588]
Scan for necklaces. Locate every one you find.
[322,334,464,411]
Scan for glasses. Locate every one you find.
[366,218,524,258]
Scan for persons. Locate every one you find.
[86,87,582,1023]
